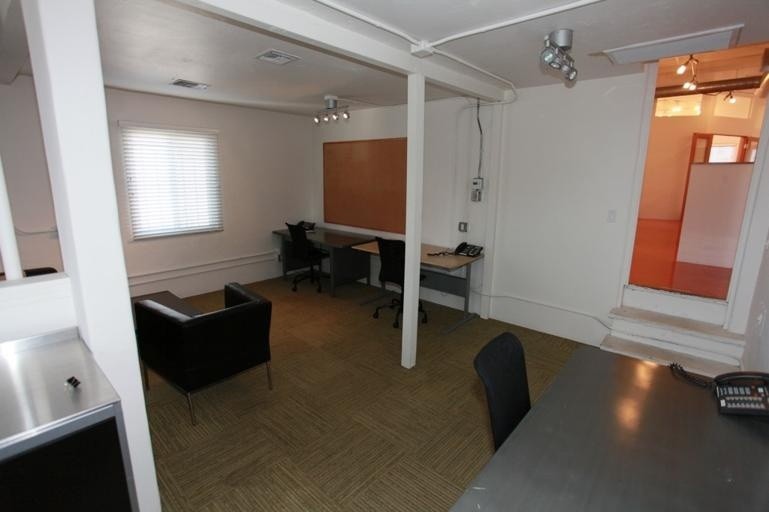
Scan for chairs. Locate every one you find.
[373,236,427,328]
[473,332,531,454]
[281,222,329,292]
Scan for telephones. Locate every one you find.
[669,362,769,416]
[298,220,316,231]
[427,242,483,257]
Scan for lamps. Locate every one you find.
[332,113,339,120]
[560,55,570,73]
[540,41,555,65]
[676,64,686,75]
[565,61,577,81]
[689,84,696,91]
[682,82,690,89]
[549,48,561,68]
[324,115,329,122]
[343,112,349,119]
[730,98,736,104]
[314,116,320,123]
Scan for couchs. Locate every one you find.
[134,282,272,426]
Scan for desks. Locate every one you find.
[449,343,769,512]
[352,239,485,333]
[272,227,380,296]
[131,291,203,317]
